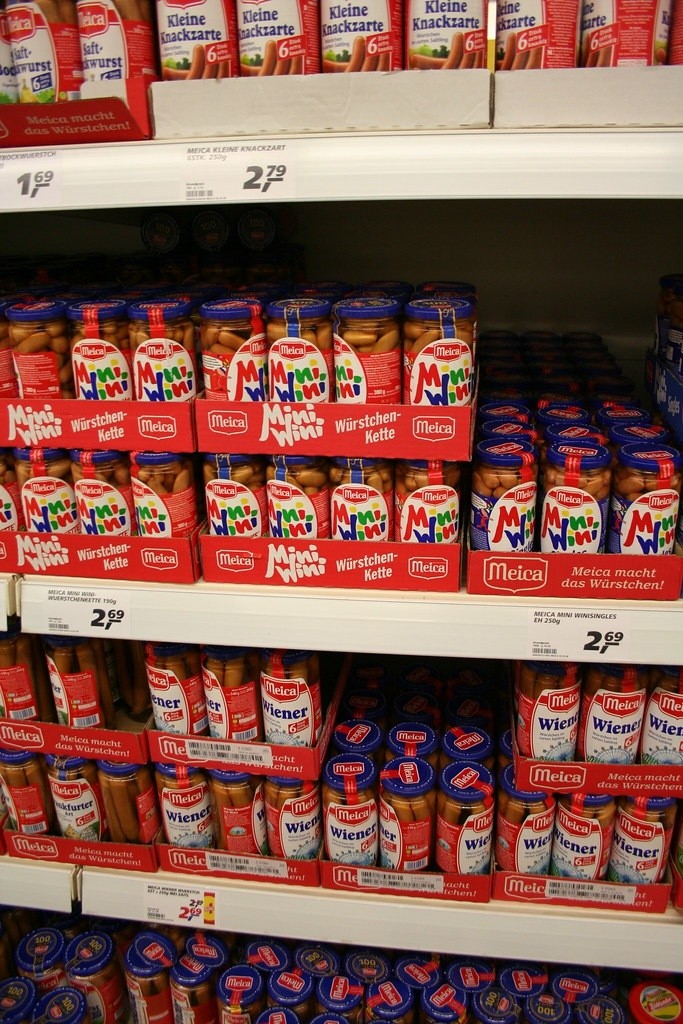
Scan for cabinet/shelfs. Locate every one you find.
[0,124,683,977]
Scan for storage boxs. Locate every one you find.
[0,14,683,921]
